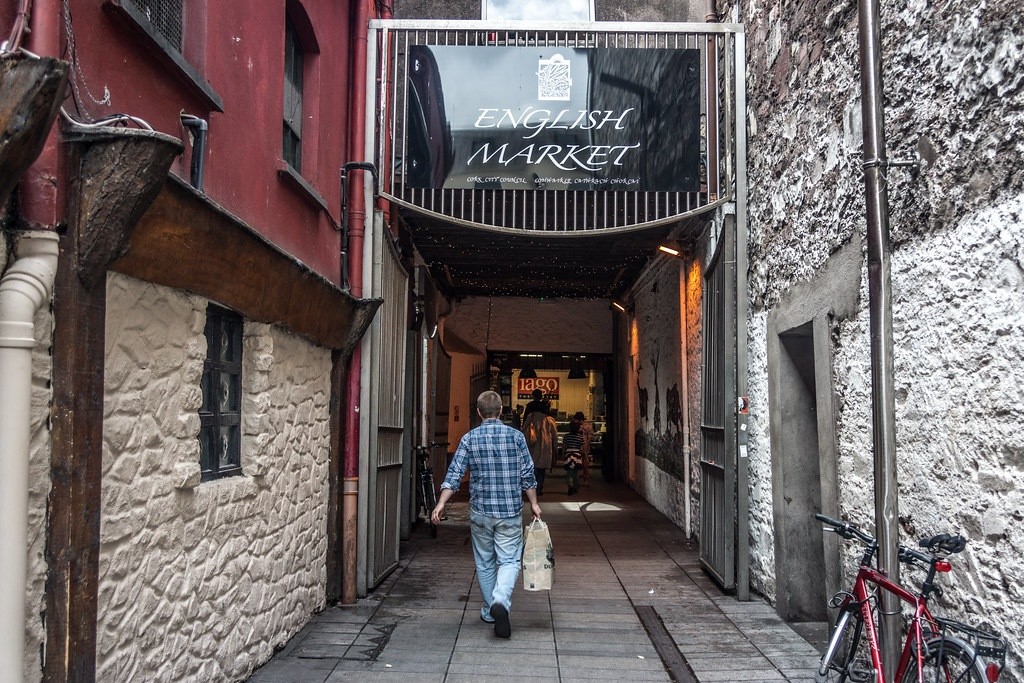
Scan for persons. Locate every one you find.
[512,389,559,497]
[431,391,544,640]
[562,411,594,497]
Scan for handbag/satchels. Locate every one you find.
[523,519,557,593]
[518,423,539,448]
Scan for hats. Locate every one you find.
[573,410,587,425]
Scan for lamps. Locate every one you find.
[658,238,696,262]
[612,298,635,314]
[519,368,538,379]
[568,368,587,379]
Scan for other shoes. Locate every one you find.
[490,603,512,639]
[565,484,578,496]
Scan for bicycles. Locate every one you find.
[411,441,450,540]
[814,510,1008,683]
